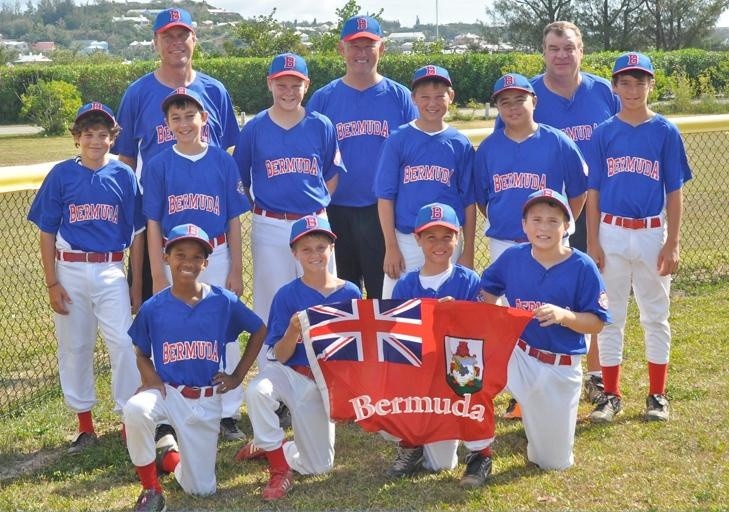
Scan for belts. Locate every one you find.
[518,339,571,365]
[57,252,123,262]
[292,365,314,379]
[163,235,225,248]
[169,383,213,398]
[255,207,325,220]
[603,215,660,229]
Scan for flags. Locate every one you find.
[296,296,536,448]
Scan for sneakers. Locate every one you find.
[156,434,179,467]
[134,490,167,512]
[262,469,292,499]
[585,375,619,422]
[502,398,520,419]
[68,432,97,455]
[461,452,491,489]
[393,447,424,474]
[220,418,266,459]
[646,393,670,421]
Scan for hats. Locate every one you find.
[75,86,204,120]
[165,224,213,254]
[270,54,309,82]
[612,52,654,76]
[152,8,193,32]
[523,189,570,221]
[412,65,536,97]
[290,215,337,245]
[415,202,460,234]
[340,17,382,42]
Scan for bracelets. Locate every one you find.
[46,280,60,288]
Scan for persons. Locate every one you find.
[137,86,250,443]
[456,188,614,487]
[305,11,421,302]
[108,7,242,306]
[23,102,149,455]
[379,203,483,478]
[473,73,588,422]
[233,53,349,429]
[373,63,476,441]
[585,51,694,425]
[231,214,360,501]
[493,19,623,404]
[121,223,268,512]
